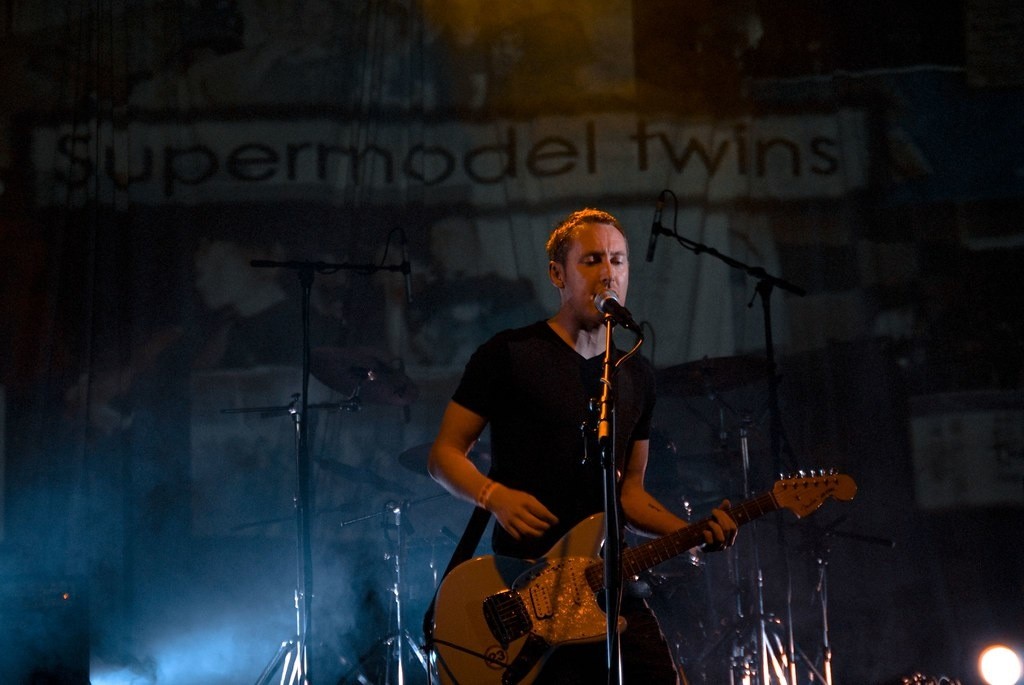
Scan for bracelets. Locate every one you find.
[477,479,498,509]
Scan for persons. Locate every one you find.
[425,208,739,685]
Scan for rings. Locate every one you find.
[719,543,727,550]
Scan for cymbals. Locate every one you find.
[399,440,492,478]
[644,424,668,477]
[317,458,416,495]
[652,354,775,397]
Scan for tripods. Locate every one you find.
[254,263,404,685]
[334,491,452,685]
[685,416,832,685]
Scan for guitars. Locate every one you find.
[433,466,858,685]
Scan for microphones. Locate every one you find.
[645,193,665,262]
[400,230,416,304]
[594,289,645,340]
